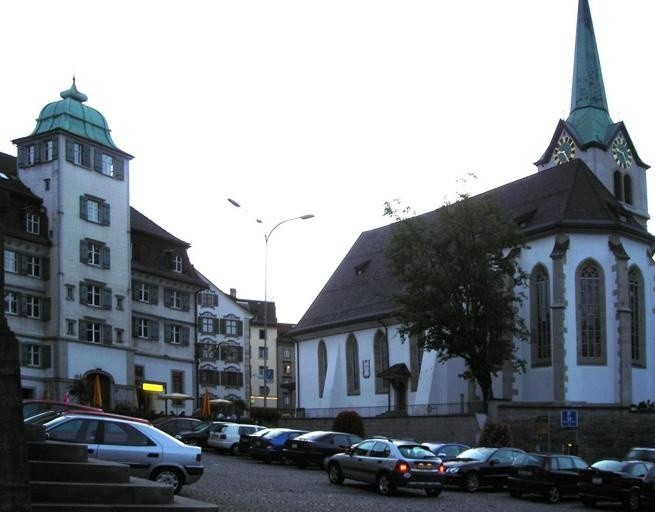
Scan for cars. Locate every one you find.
[507,451,592,503]
[326,438,446,496]
[574,458,655,512]
[239,428,276,454]
[20,398,154,446]
[175,420,232,447]
[624,446,655,462]
[640,463,655,511]
[28,412,205,496]
[249,427,311,466]
[283,430,365,470]
[418,440,472,463]
[206,423,267,455]
[152,414,202,437]
[442,446,529,493]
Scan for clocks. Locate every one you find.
[610,136,632,170]
[554,137,576,167]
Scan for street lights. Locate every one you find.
[227,196,316,425]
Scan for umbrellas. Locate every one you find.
[201,386,211,417]
[209,399,232,412]
[162,392,196,415]
[93,374,102,408]
